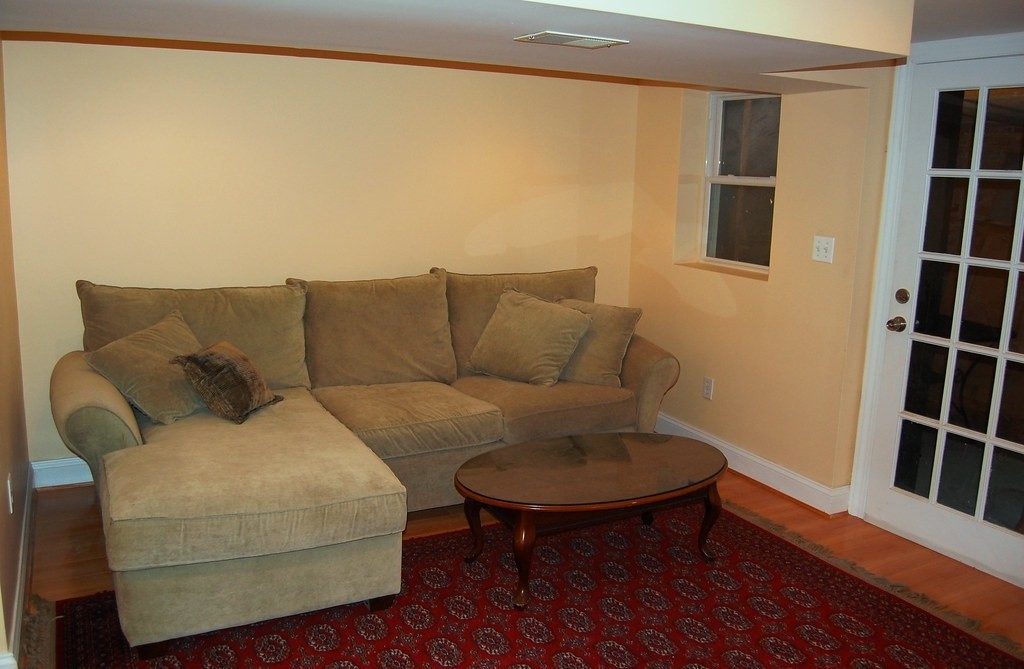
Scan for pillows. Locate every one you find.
[82,308,285,425]
[465,284,645,388]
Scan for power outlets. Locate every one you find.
[701,376,714,400]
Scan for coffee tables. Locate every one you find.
[454,431,731,608]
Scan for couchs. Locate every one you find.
[50,264,679,645]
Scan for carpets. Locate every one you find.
[27,492,1024,669]
